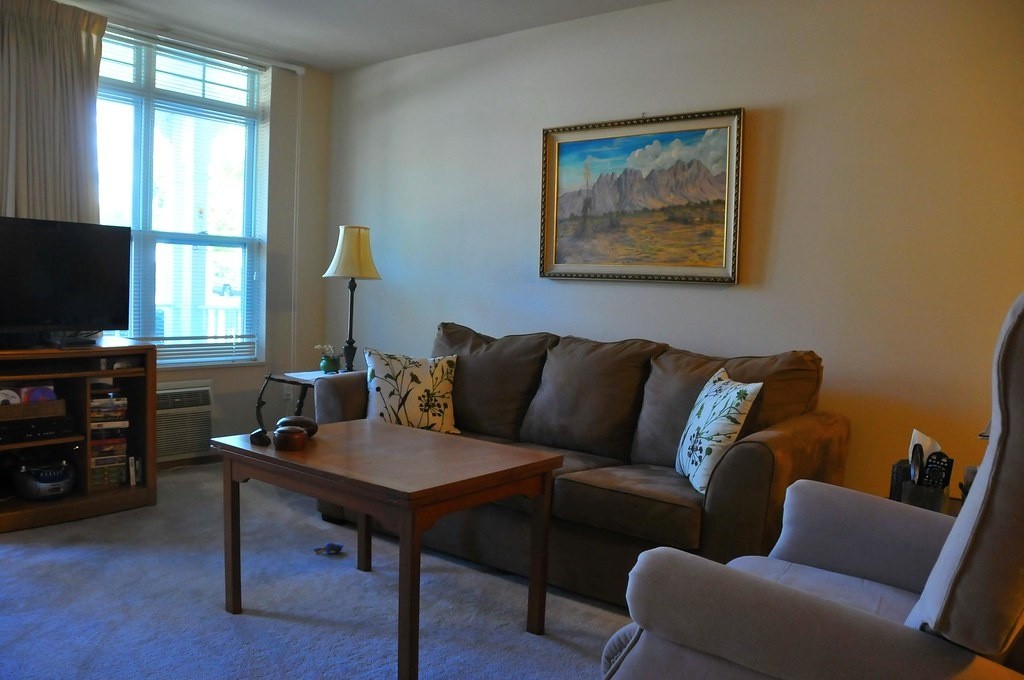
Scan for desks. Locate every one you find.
[265,370,347,416]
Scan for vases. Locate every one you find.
[320,356,341,374]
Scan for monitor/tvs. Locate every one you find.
[0,216,130,348]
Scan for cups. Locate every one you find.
[903,480,915,499]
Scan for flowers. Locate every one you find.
[314,344,340,360]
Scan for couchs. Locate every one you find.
[315,373,851,608]
[602,288,1024,680]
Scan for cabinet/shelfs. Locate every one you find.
[0,336,158,533]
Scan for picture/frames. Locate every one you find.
[540,108,744,286]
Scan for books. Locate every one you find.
[91,399,128,428]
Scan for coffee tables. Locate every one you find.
[210,417,565,680]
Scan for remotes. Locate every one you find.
[909,444,949,488]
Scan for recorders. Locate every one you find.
[14,460,76,500]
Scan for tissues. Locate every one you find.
[888,427,942,501]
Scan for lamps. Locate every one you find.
[322,226,382,373]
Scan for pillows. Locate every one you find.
[363,348,461,435]
[675,367,765,494]
[517,336,670,462]
[629,347,824,466]
[431,323,558,438]
